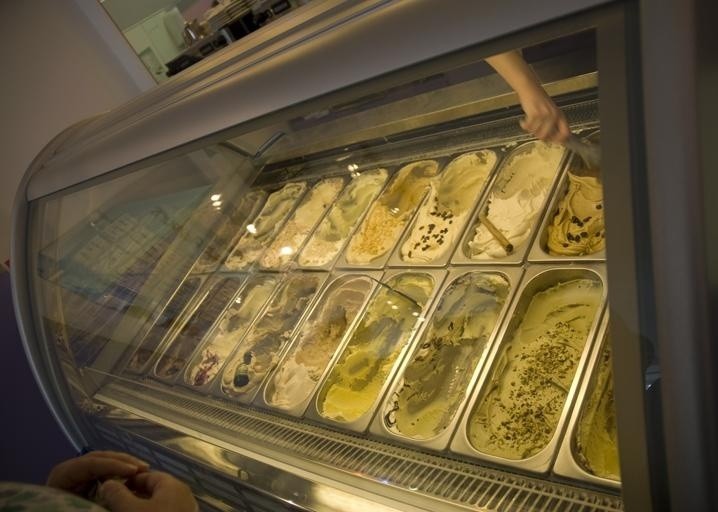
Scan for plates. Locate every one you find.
[203,0,257,30]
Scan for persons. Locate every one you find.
[0,448,201,512]
[483,48,572,146]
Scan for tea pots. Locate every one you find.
[180,18,208,45]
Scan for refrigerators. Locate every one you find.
[7,0,716,512]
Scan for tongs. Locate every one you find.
[518,110,601,174]
